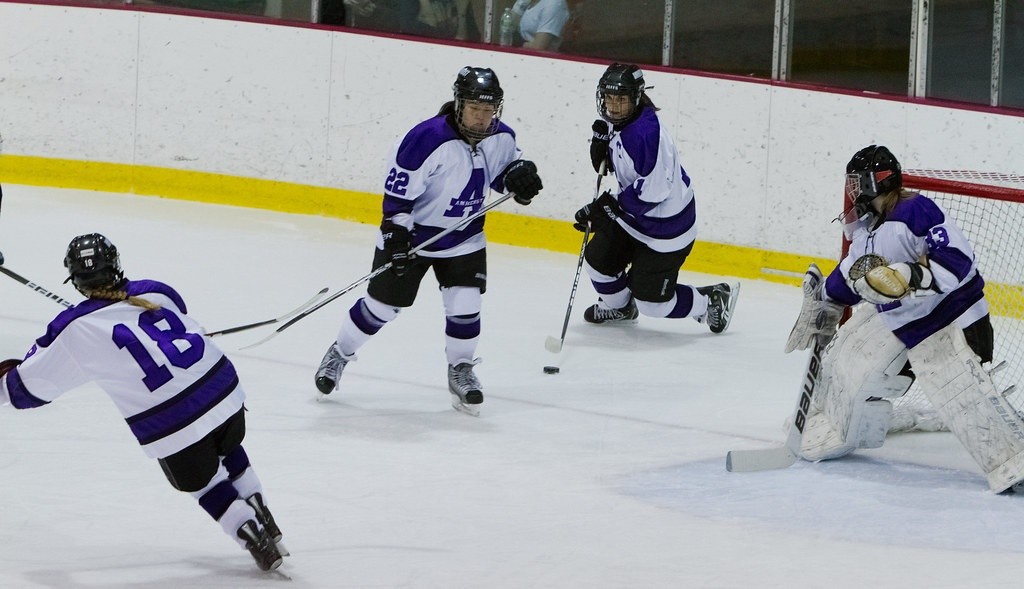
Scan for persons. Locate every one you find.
[784,146,1024,493]
[316,67,545,418]
[344,0,570,54]
[572,62,741,334]
[1,234,294,581]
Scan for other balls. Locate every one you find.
[543,366,559,373]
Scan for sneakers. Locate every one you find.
[447,357,484,417]
[314,340,357,402]
[237,519,294,582]
[695,281,740,334]
[245,492,291,556]
[584,293,639,326]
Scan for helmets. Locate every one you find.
[599,62,645,106]
[64,233,117,286]
[454,66,504,111]
[846,145,902,204]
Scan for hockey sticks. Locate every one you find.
[543,159,612,354]
[724,336,825,474]
[0,266,73,308]
[238,190,519,353]
[205,285,330,340]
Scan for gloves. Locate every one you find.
[504,159,543,205]
[379,220,420,276]
[573,191,625,233]
[590,119,614,176]
[853,261,933,304]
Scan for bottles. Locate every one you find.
[500,8,514,46]
